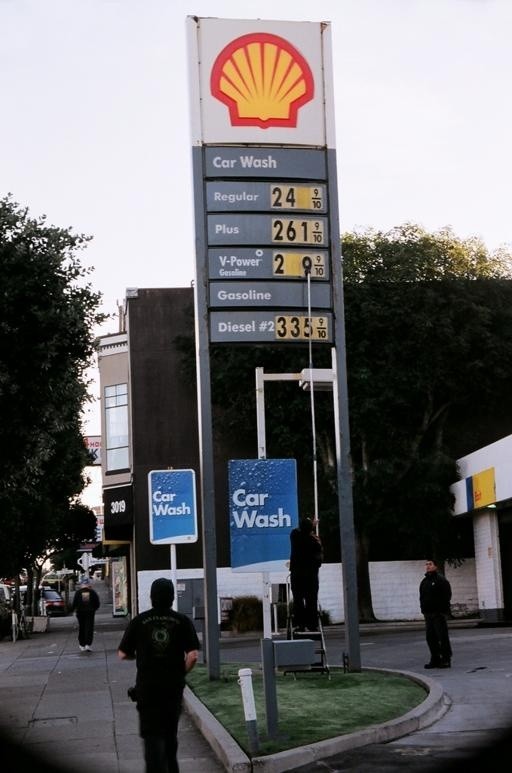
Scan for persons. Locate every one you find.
[418,558,454,669]
[116,576,201,772]
[288,514,324,633]
[66,575,100,651]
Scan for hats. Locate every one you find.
[150,578,174,602]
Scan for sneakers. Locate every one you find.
[424,658,451,669]
[295,625,318,632]
[79,644,92,652]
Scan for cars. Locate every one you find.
[0,568,103,639]
